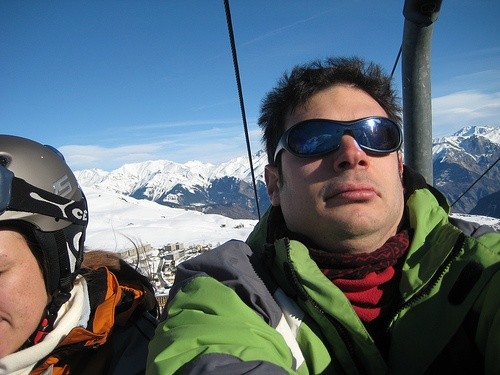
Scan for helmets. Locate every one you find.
[1,134,88,299]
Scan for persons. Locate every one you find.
[146,57,499,375]
[1,134,163,375]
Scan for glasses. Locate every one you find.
[273,116,404,167]
